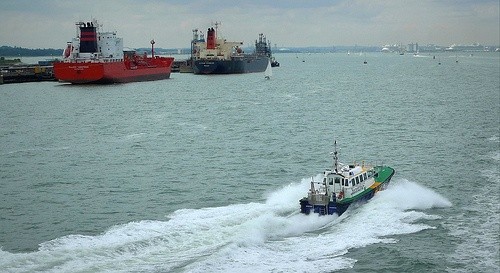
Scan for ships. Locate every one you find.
[191,20,272,74]
[53,18,175,86]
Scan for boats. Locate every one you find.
[271,57,280,67]
[301,139,396,217]
[294,49,477,70]
